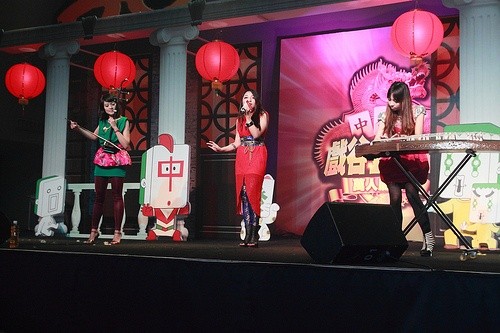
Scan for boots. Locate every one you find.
[239,225,251,247]
[247,225,261,246]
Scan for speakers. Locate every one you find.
[300,201,408,265]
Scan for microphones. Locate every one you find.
[241,108,246,114]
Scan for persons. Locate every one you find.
[370,82,435,257]
[69,93,131,245]
[206,89,270,248]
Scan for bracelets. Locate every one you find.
[246,121,253,127]
[114,129,120,133]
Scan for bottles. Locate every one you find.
[9,220,20,249]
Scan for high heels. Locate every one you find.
[420,231,435,256]
[83,230,100,244]
[108,232,122,246]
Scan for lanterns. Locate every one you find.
[94,49,136,94]
[391,8,444,65]
[6,62,46,103]
[196,39,240,88]
